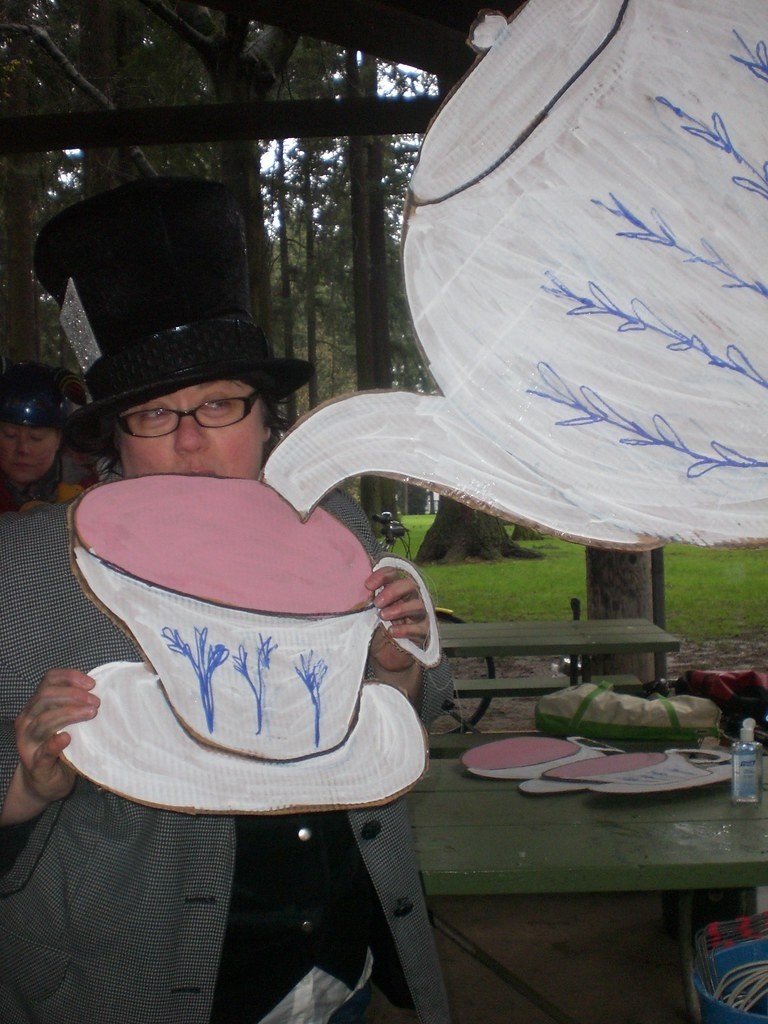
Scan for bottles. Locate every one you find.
[731,717,763,808]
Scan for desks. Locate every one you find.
[429,618,681,701]
[407,753,768,1023]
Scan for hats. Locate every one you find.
[33,174,316,423]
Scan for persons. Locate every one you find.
[0,172,456,1024]
[0,355,87,514]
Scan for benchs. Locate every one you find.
[426,730,735,762]
[445,675,646,701]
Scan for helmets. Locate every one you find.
[0,360,70,428]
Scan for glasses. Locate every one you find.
[114,389,260,439]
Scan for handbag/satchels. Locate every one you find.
[674,669,768,720]
[533,680,720,740]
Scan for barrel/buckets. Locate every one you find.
[693,935,768,1024]
